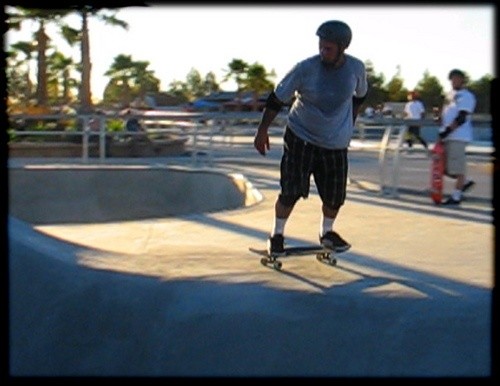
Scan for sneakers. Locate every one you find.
[268,234,285,256]
[442,197,463,205]
[462,181,474,193]
[319,231,349,251]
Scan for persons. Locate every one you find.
[253,20,369,257]
[403,92,429,152]
[438,69,477,207]
[126,110,161,151]
[83,111,112,158]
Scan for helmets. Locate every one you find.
[449,69,468,83]
[316,21,352,48]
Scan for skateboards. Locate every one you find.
[423,140,445,204]
[248,245,351,270]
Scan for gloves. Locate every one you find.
[438,127,453,139]
